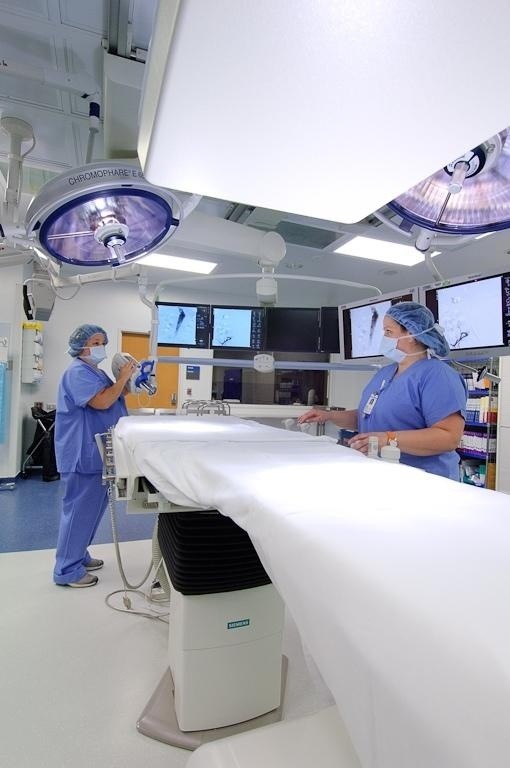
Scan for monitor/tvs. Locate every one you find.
[266,309,320,353]
[321,306,340,353]
[155,302,210,350]
[419,269,509,359]
[211,305,266,353]
[338,287,419,363]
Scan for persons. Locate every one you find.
[53,323,140,588]
[297,300,470,486]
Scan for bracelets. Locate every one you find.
[386,429,400,448]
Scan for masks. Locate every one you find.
[81,345,107,367]
[378,334,415,363]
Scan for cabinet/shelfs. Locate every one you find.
[22,329,43,383]
[448,355,498,489]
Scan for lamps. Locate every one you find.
[371,127,510,254]
[24,161,184,270]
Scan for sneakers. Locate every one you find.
[83,557,104,572]
[56,572,98,589]
